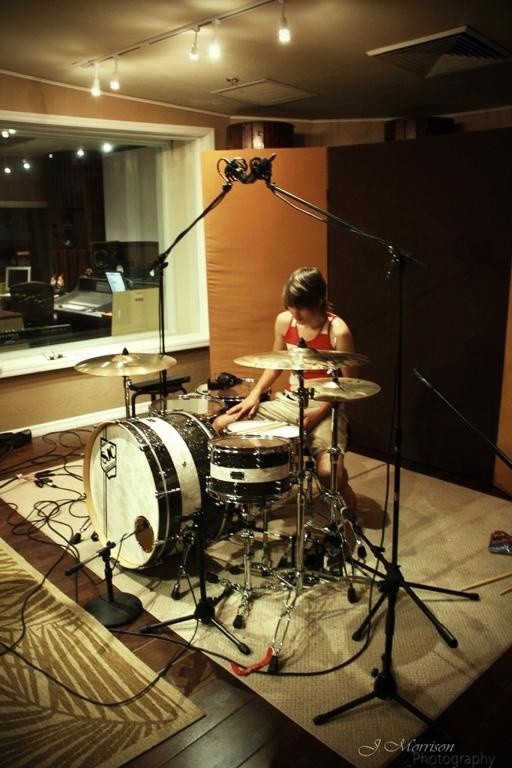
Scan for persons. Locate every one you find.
[212,266,359,513]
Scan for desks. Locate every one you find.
[52,277,160,328]
[0,307,24,332]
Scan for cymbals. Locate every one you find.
[231,354,372,374]
[73,352,173,376]
[292,378,378,407]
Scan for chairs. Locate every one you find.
[9,280,99,332]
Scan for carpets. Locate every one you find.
[0,450,511,767]
[0,534,206,766]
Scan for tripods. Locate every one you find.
[313,277,461,746]
[227,503,295,572]
[206,523,291,628]
[263,490,338,675]
[352,264,480,649]
[140,520,250,656]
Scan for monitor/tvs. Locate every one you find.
[5,266,31,291]
[89,241,120,276]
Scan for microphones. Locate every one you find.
[222,158,247,189]
[245,153,276,183]
[176,505,217,522]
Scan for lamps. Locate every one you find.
[90,63,102,97]
[207,17,222,59]
[277,1,292,45]
[188,25,200,61]
[109,54,121,92]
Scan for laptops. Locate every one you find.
[92,270,129,314]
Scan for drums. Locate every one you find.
[82,417,220,569]
[225,413,301,451]
[148,396,228,420]
[207,437,295,505]
[198,377,273,408]
